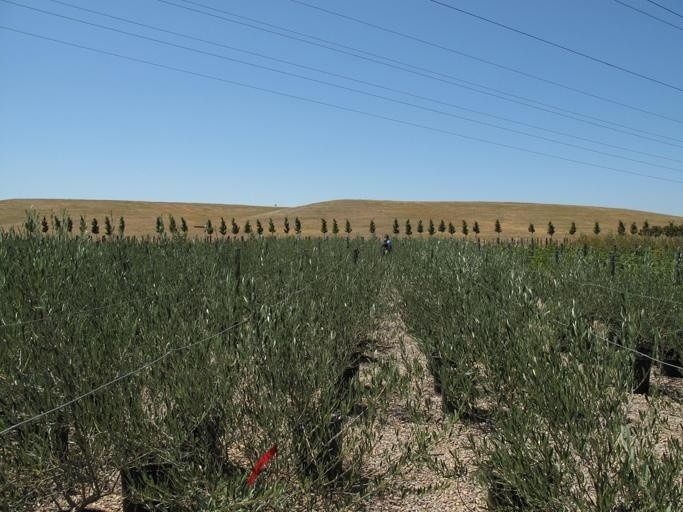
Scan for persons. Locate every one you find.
[382,234,392,255]
